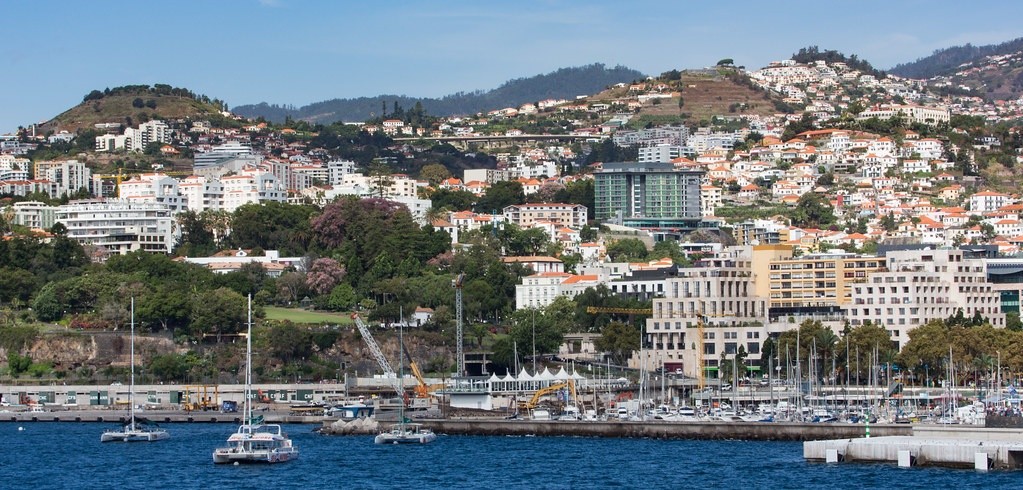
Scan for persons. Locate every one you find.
[987,400,1023,418]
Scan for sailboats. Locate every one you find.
[99,297,1023,463]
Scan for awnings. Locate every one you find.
[484,366,585,391]
